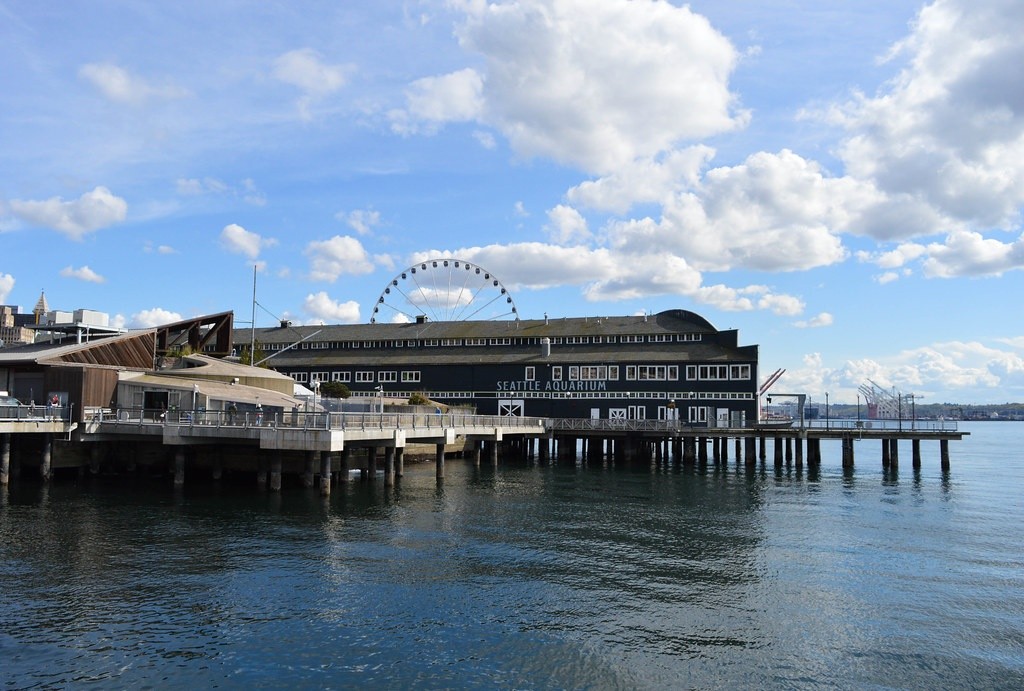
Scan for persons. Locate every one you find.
[255,403,263,426]
[228,402,238,426]
[435,407,450,414]
[52,394,59,406]
[160,402,166,424]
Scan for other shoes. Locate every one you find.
[53,420,56,423]
[48,420,51,422]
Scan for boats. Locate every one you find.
[760,408,793,421]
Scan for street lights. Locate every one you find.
[825,392,829,431]
[910,392,916,431]
[310,379,321,428]
[566,391,571,416]
[626,390,630,420]
[690,392,693,428]
[808,394,812,427]
[898,391,902,432]
[857,394,860,428]
[510,391,514,416]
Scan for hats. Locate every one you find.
[670,400,675,403]
[231,401,236,404]
[257,403,261,408]
